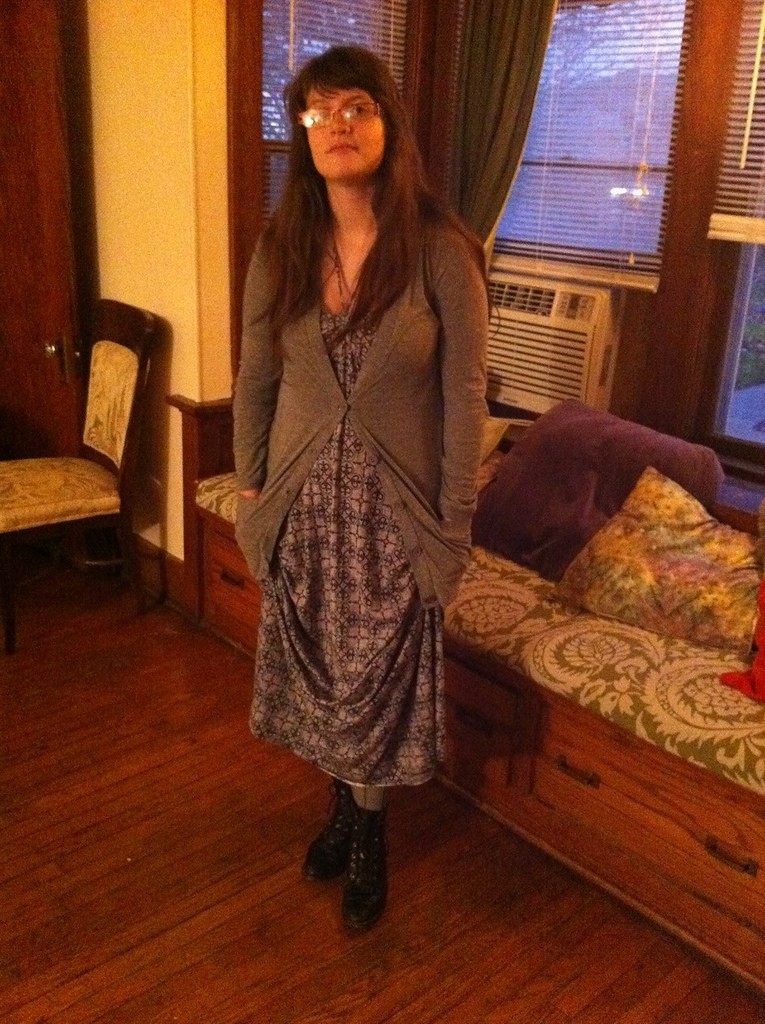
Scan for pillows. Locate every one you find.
[720,587,764,703]
[471,399,727,582]
[548,465,761,660]
[479,416,511,464]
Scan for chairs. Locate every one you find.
[0,297,153,654]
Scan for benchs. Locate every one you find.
[191,399,765,989]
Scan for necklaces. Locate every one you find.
[320,219,369,316]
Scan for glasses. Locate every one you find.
[299,102,386,128]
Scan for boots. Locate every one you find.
[343,801,389,928]
[301,778,350,883]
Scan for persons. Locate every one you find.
[231,43,497,932]
